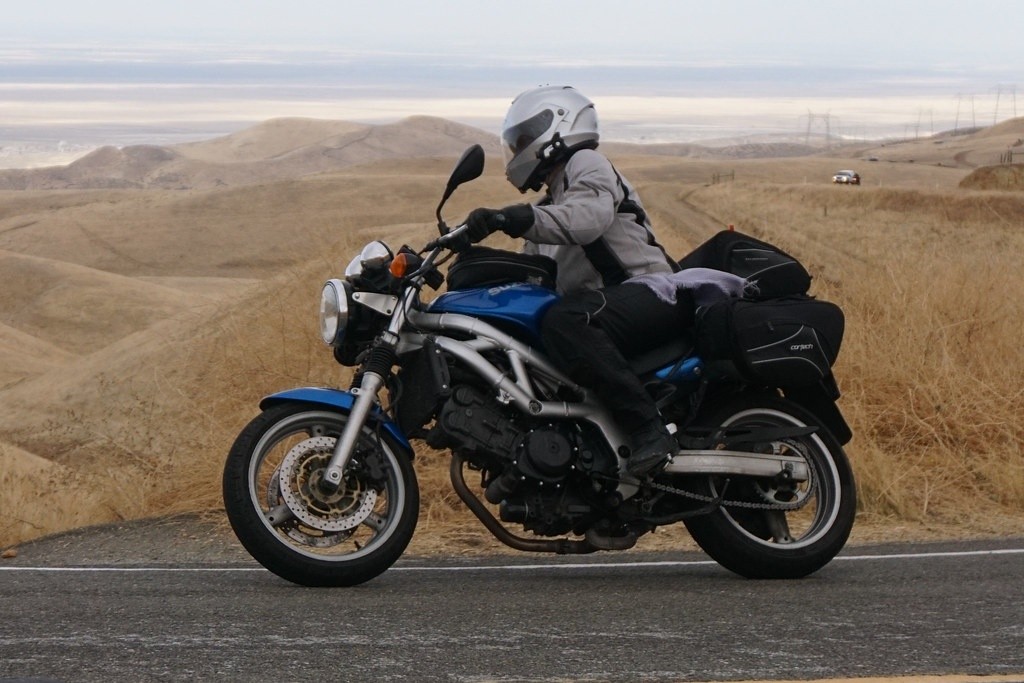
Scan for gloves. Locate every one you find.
[456,202,536,244]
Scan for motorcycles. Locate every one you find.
[221,143,860,586]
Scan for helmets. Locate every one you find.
[501,83,600,195]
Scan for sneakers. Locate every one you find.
[626,414,681,478]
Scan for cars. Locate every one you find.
[832,169,862,186]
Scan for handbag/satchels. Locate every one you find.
[446,244,559,293]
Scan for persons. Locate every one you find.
[462,86,689,473]
